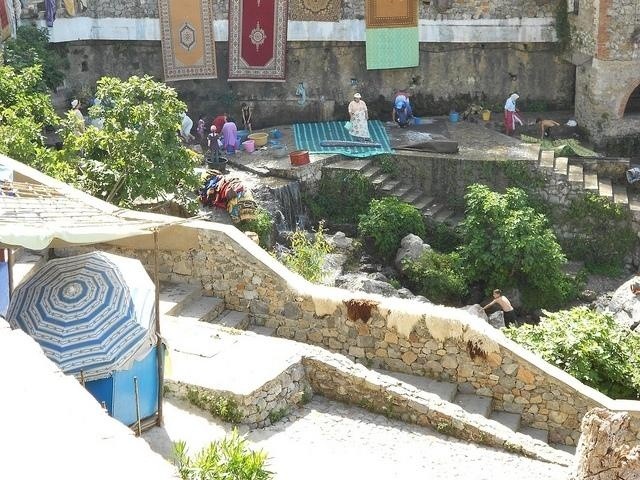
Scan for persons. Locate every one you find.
[479,287,518,329]
[220,121,238,156]
[178,112,195,142]
[347,91,371,140]
[391,91,417,128]
[207,125,223,163]
[197,112,208,143]
[503,93,522,137]
[240,102,255,132]
[534,117,561,139]
[210,112,230,130]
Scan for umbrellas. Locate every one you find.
[4,247,159,387]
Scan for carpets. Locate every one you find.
[292,118,395,158]
[158,1,219,83]
[362,0,420,71]
[226,1,289,84]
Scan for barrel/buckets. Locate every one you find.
[415,117,421,124]
[344,119,354,131]
[482,109,491,121]
[450,110,461,124]
[243,140,255,153]
